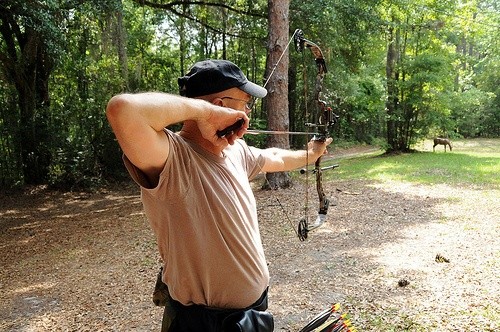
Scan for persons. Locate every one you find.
[107,60,333,332]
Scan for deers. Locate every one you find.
[433,138,452,152]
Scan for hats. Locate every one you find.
[176,60,268,101]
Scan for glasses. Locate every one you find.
[219,96,256,109]
